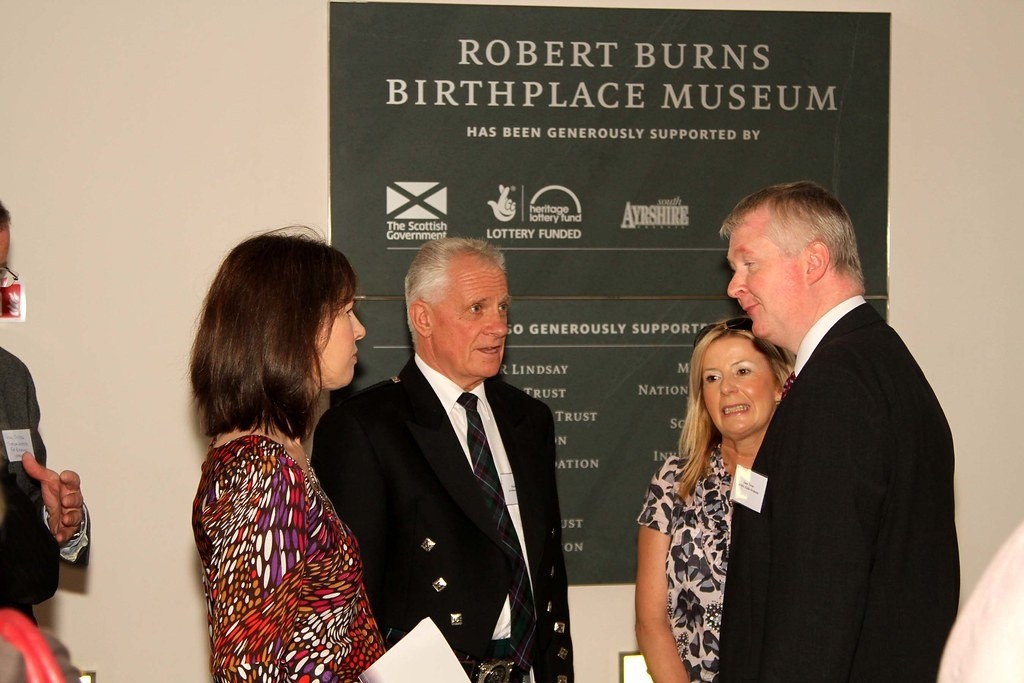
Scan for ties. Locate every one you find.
[781,370,797,401]
[456,390,539,671]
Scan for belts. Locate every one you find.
[456,651,525,683]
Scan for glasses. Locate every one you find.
[0,265,19,288]
[693,318,787,367]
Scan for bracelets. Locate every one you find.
[73,519,85,538]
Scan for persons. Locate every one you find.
[636,316,796,683]
[310,238,576,683]
[718,182,961,683]
[190,234,387,683]
[0,200,91,628]
